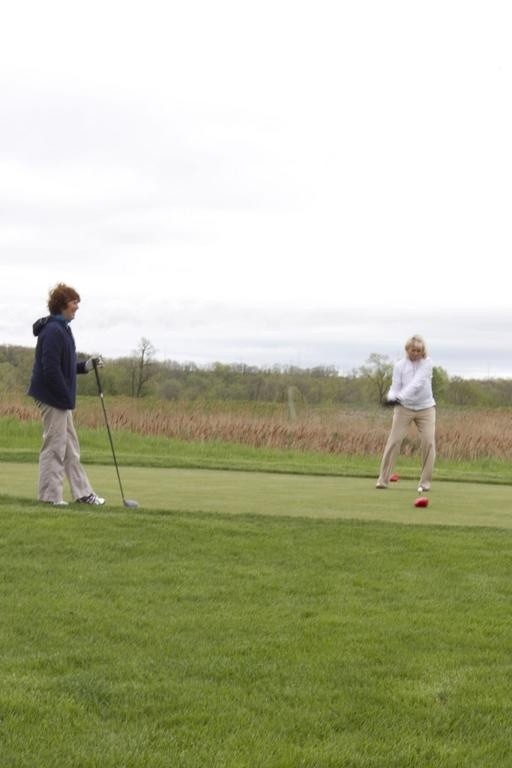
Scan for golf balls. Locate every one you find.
[418,487,423,492]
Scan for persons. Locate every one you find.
[375,336,437,492]
[26,283,105,507]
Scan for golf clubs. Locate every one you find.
[93,360,138,508]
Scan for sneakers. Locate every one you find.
[74,491,106,507]
[36,500,68,507]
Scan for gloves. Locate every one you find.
[85,356,104,371]
